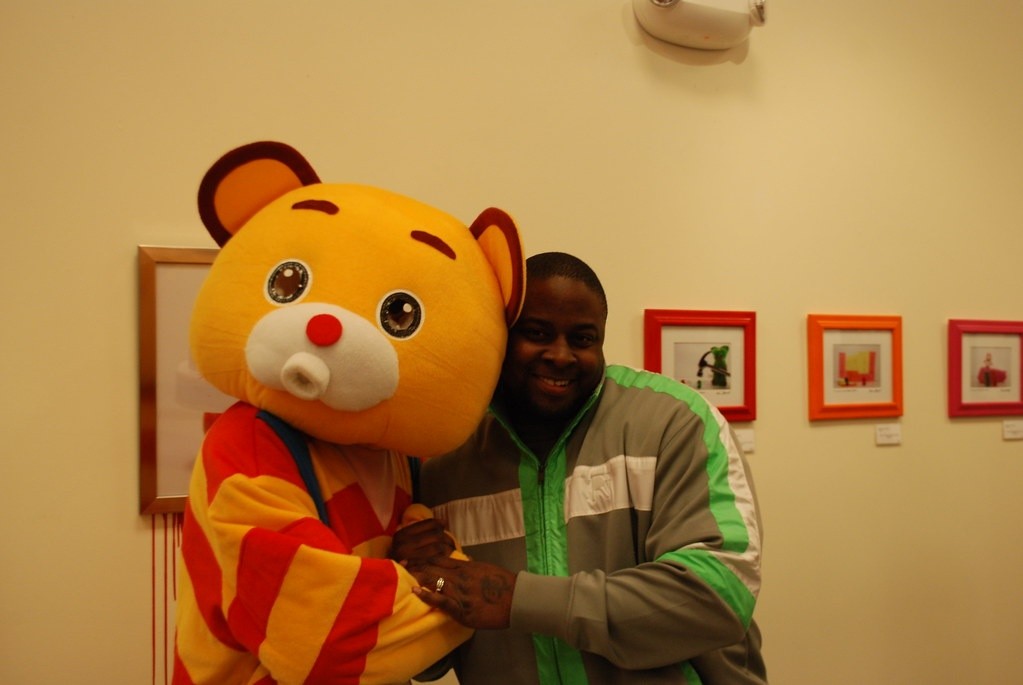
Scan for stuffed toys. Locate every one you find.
[172,141,526,685]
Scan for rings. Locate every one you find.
[437,576,445,593]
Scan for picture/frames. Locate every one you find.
[643,308,756,422]
[948,319,1023,417]
[806,314,903,421]
[136,246,221,515]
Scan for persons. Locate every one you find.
[385,251,768,685]
